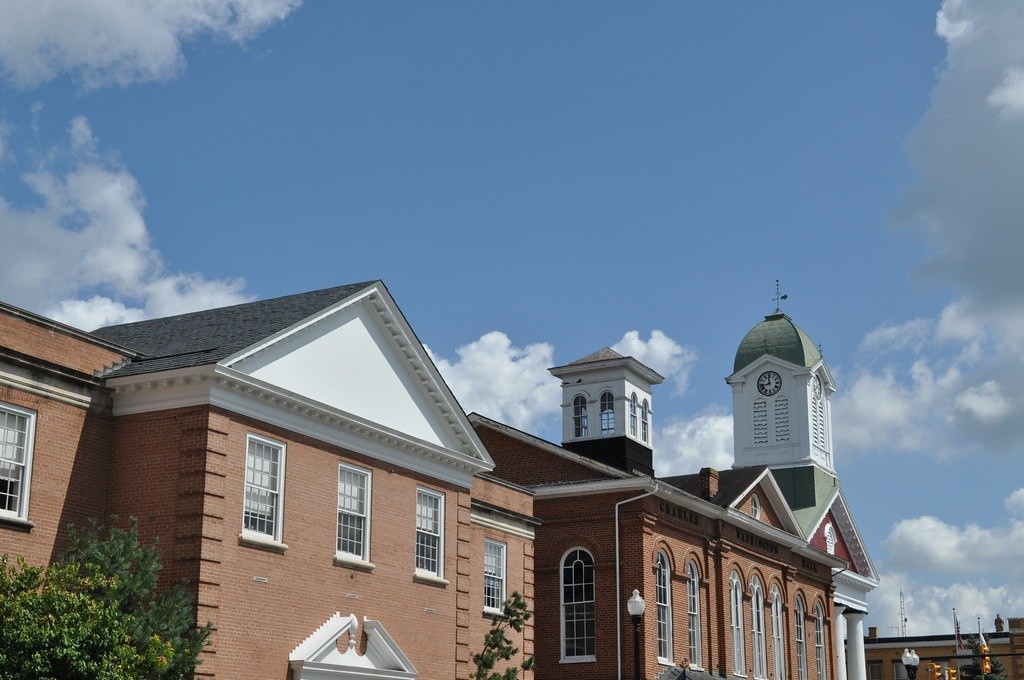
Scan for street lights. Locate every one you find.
[627,589,646,680]
[901,648,920,680]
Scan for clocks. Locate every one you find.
[814,374,822,400]
[757,371,782,397]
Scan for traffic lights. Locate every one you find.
[948,666,958,680]
[980,643,991,674]
[928,662,942,680]
